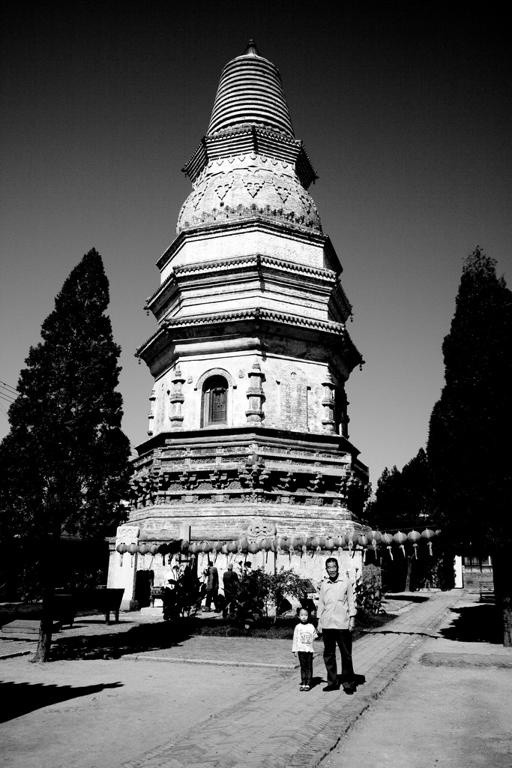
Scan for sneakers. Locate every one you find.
[323,685,339,690]
[344,688,353,694]
[300,685,310,690]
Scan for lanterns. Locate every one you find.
[117,528,434,568]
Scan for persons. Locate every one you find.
[292,608,319,691]
[315,557,358,692]
[169,559,252,613]
